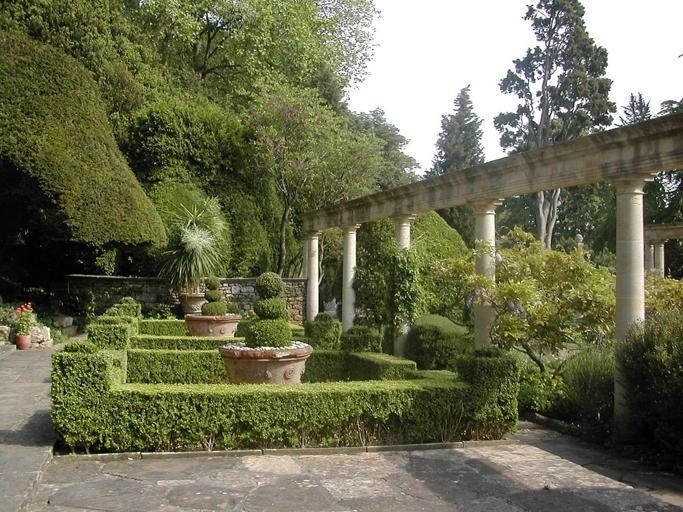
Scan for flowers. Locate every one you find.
[13,303,35,335]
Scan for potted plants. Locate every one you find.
[217,272,313,384]
[157,228,225,315]
[183,275,242,339]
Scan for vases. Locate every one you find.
[16,335,32,350]
